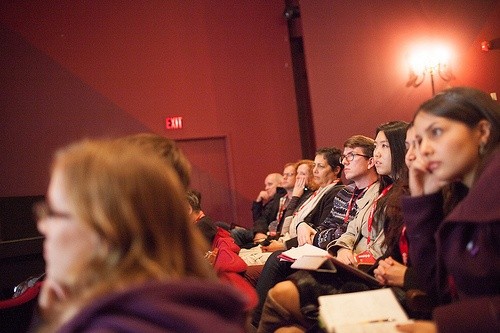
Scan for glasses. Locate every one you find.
[34,202,70,221]
[338,152,371,164]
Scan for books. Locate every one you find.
[318,288,411,333]
[278,243,384,285]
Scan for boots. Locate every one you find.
[257,290,292,333]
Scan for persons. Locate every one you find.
[232,121,431,333]
[37,137,251,333]
[396,86,500,333]
[120,134,218,257]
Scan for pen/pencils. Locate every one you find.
[368,318,396,323]
